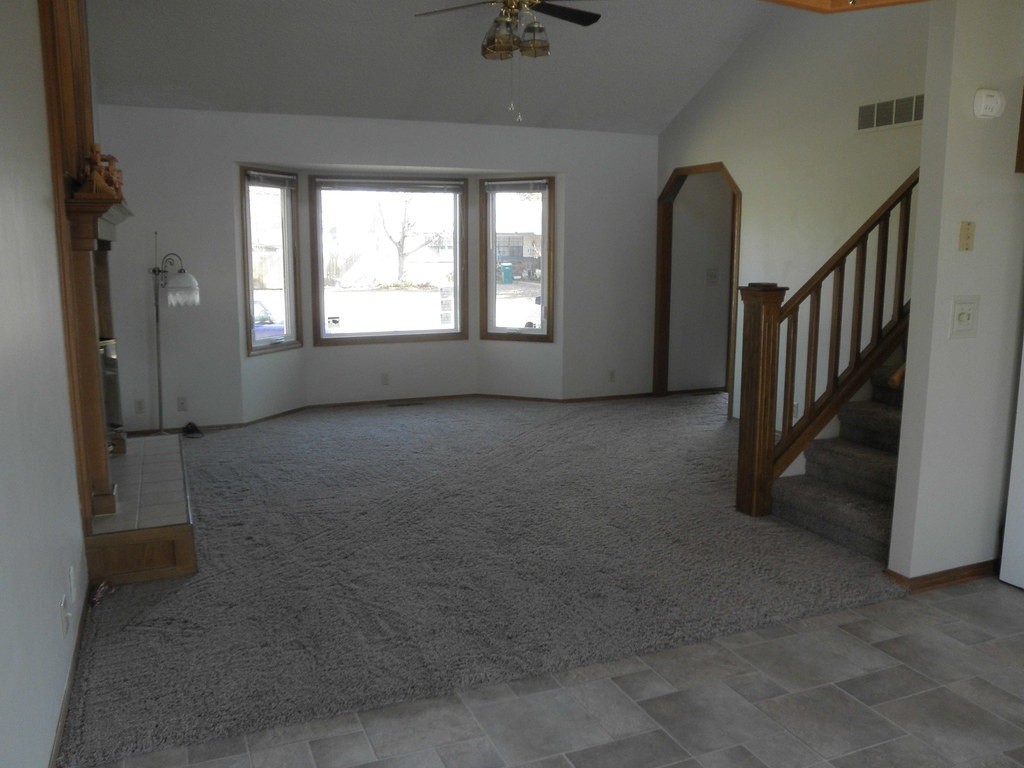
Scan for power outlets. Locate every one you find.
[177,396,188,411]
[135,399,145,413]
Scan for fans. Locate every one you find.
[413,0,601,29]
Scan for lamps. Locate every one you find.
[480,0,552,60]
[150,231,202,433]
